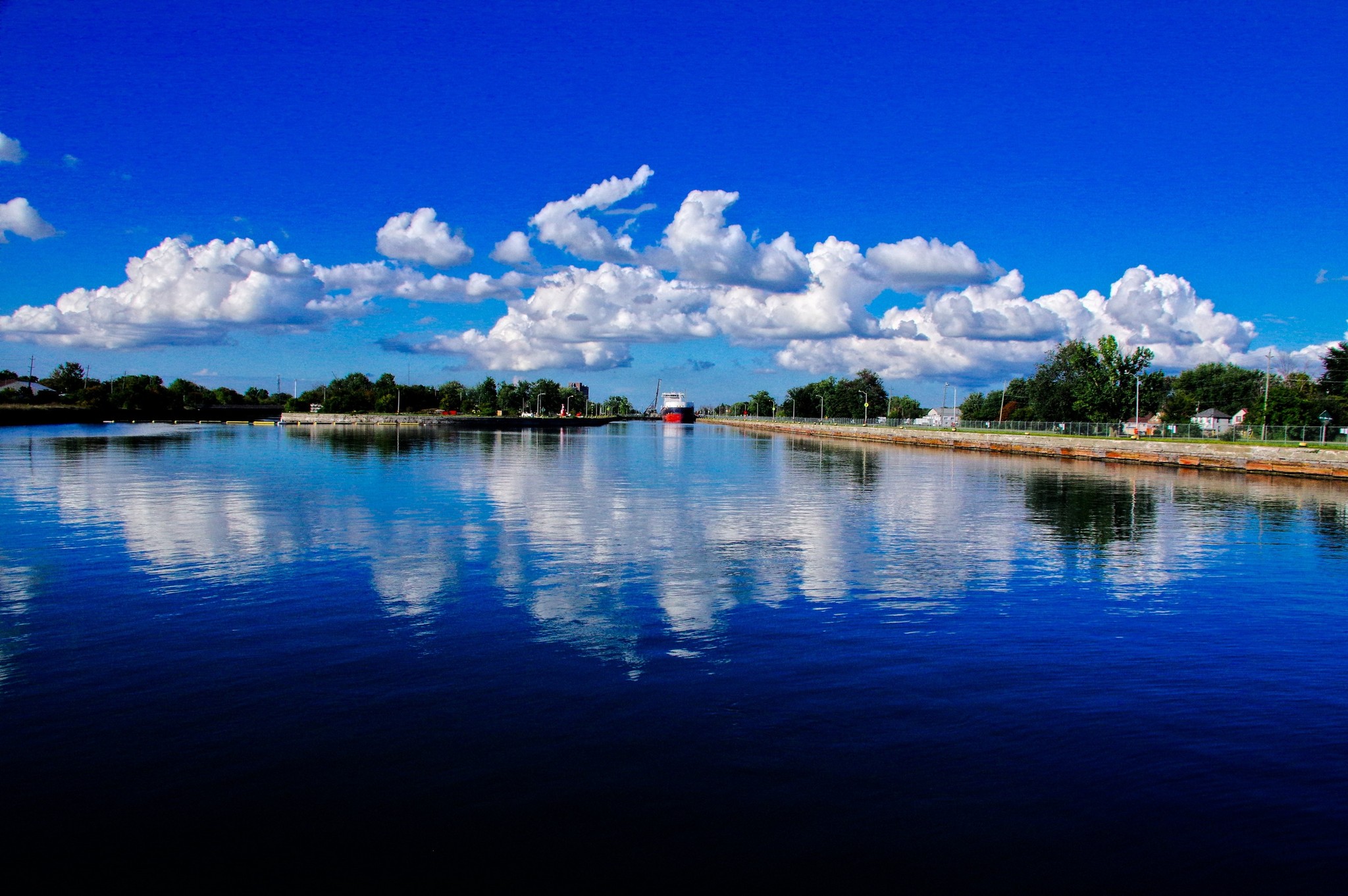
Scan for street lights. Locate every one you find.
[786,398,795,421]
[941,383,950,427]
[567,395,574,416]
[537,393,546,415]
[1124,372,1138,431]
[858,390,867,424]
[397,388,400,414]
[816,395,823,422]
[743,403,746,420]
[755,402,758,420]
[768,400,774,419]
[734,405,736,418]
[592,398,614,417]
[586,398,592,417]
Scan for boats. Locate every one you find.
[659,389,697,424]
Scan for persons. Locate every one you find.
[988,420,991,429]
[863,420,867,427]
[820,420,823,424]
